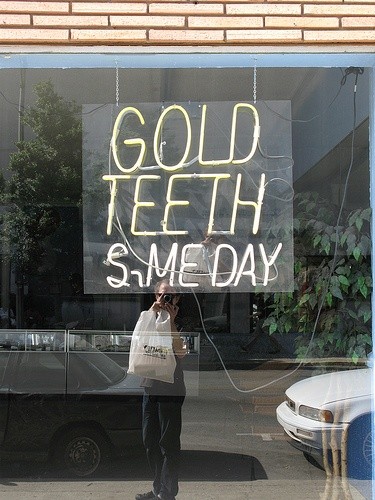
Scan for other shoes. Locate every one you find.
[135,491,154,500]
[148,496,176,500]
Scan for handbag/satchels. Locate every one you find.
[126,304,176,386]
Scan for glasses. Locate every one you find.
[157,293,178,298]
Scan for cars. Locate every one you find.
[275,338,373,479]
[0,329,173,479]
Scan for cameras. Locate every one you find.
[162,293,173,305]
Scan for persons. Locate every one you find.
[137,279,189,500]
